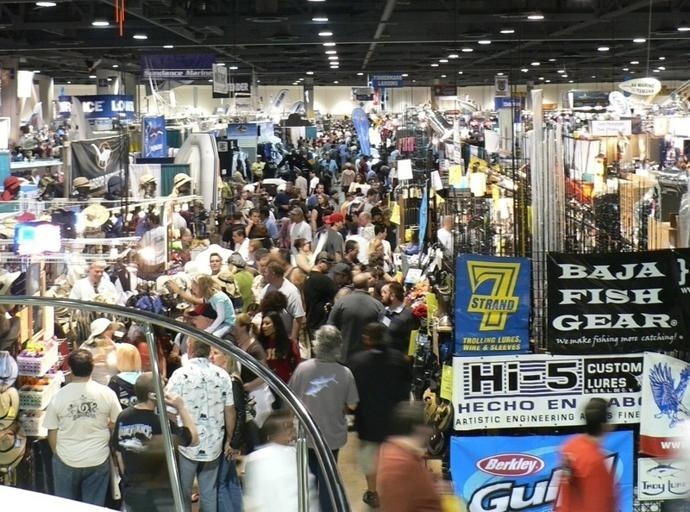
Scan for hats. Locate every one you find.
[38,177,55,186]
[226,256,246,268]
[0,270,23,294]
[0,350,27,472]
[173,173,192,188]
[138,176,159,186]
[108,176,124,194]
[88,317,111,337]
[74,176,92,187]
[211,271,241,295]
[324,212,344,223]
[80,204,110,228]
[316,252,350,275]
[3,178,25,189]
[291,207,304,215]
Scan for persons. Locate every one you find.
[0,102,690,512]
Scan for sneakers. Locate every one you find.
[363,491,378,507]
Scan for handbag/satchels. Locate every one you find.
[216,454,243,511]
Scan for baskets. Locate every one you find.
[18,341,59,376]
[19,371,63,410]
[17,412,48,437]
